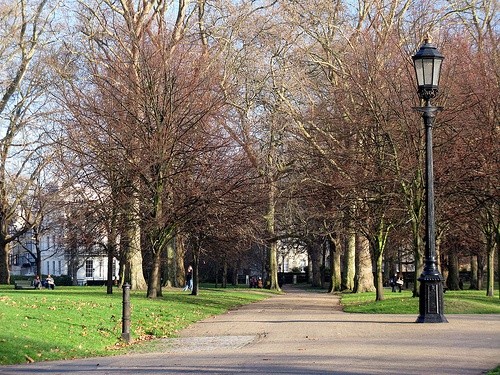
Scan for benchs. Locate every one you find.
[14,279,35,290]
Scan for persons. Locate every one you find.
[390,273,403,293]
[316,271,321,286]
[279,276,286,289]
[34,274,55,290]
[459,278,463,289]
[114,277,118,286]
[249,276,263,288]
[183,266,193,291]
[161,276,171,288]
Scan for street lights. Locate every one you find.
[410,30,448,324]
